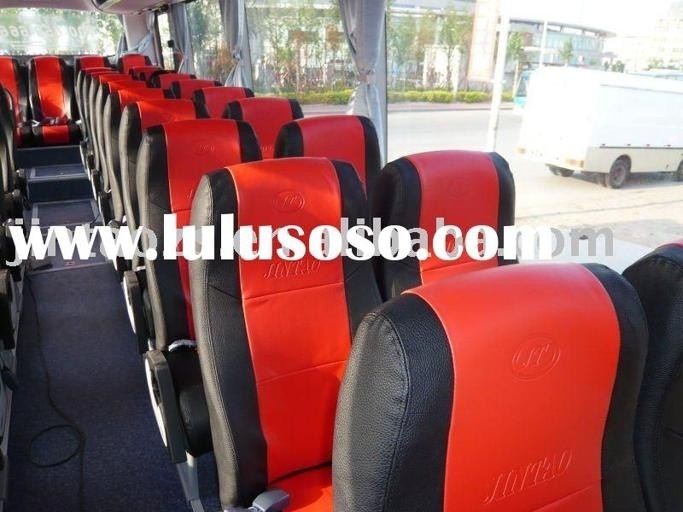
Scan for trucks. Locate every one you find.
[516,63,683,189]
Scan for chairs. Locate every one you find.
[29,55,78,146]
[0,55,31,512]
